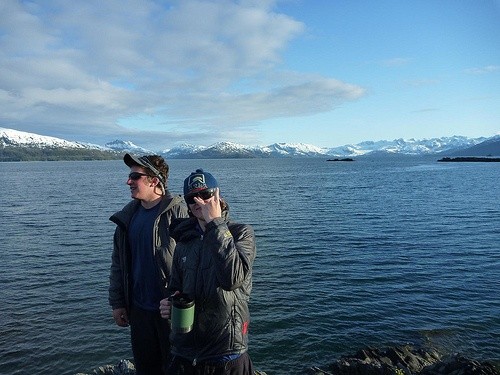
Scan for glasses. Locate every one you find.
[128,172,151,180]
[184,192,216,204]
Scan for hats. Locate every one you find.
[183,168,218,197]
[123,153,165,185]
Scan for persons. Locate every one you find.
[109,154,190,375]
[159,167,257,375]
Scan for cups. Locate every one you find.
[167,293,195,334]
